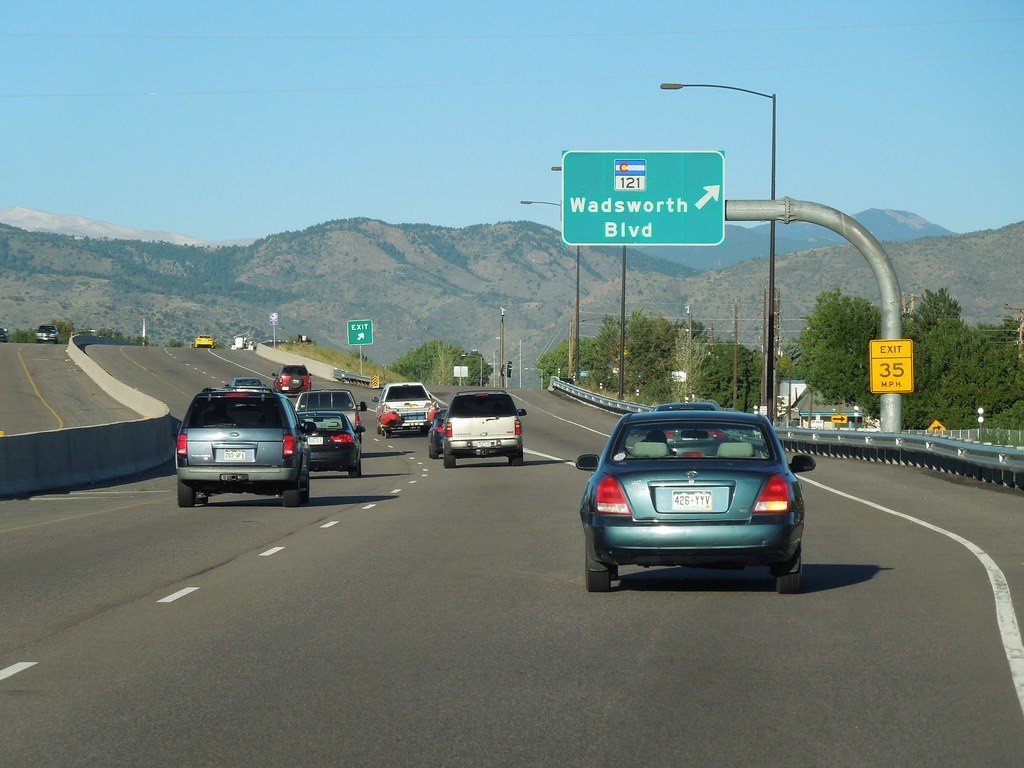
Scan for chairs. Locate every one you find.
[327,423,338,428]
[636,441,670,458]
[718,442,755,458]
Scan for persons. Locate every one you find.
[639,429,677,456]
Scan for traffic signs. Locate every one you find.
[346,319,374,345]
[560,151,725,247]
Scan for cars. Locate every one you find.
[35,324,60,344]
[291,411,365,478]
[273,364,313,395]
[173,377,311,508]
[575,408,813,596]
[439,391,527,467]
[194,334,216,350]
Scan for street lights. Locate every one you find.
[551,166,627,400]
[522,199,582,386]
[660,83,778,432]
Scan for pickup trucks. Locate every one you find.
[294,389,362,440]
[372,382,438,437]
